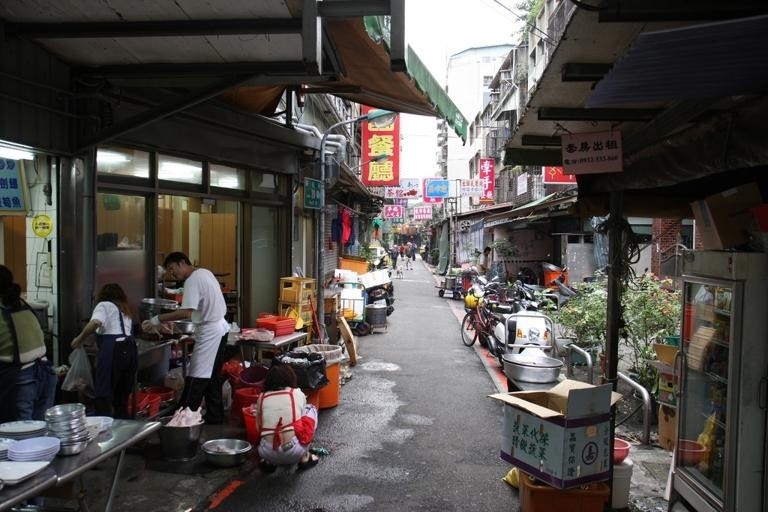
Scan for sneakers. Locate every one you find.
[201,413,224,425]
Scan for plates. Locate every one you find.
[0,419,61,486]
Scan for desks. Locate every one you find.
[237,331,308,368]
[0,416,162,512]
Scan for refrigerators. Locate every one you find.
[670,247,767,512]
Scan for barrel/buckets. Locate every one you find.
[612,457,634,509]
[366,302,387,334]
[236,388,262,426]
[157,417,204,463]
[243,406,261,444]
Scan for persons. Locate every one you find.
[71,283,137,418]
[141,251,231,426]
[2,262,56,507]
[412,242,417,261]
[405,241,414,270]
[476,247,492,272]
[252,362,320,470]
[390,244,399,267]
[399,243,405,261]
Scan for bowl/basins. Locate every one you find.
[144,386,177,409]
[85,416,115,440]
[500,353,564,384]
[200,438,252,467]
[678,439,706,466]
[43,402,92,457]
[613,437,631,466]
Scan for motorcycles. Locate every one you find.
[385,249,400,270]
[367,256,395,317]
[458,264,598,370]
[418,245,426,260]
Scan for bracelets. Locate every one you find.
[150,313,161,327]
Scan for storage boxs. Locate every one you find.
[487,377,623,511]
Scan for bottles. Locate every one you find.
[709,434,725,490]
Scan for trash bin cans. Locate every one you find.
[273,349,325,413]
[299,344,342,410]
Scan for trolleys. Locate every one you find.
[334,280,371,339]
[435,274,463,300]
[501,310,567,392]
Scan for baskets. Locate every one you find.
[464,292,484,308]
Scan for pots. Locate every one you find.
[137,297,178,342]
[365,303,387,329]
[444,276,455,290]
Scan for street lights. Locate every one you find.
[416,225,428,262]
[339,151,390,261]
[444,195,460,274]
[315,107,400,344]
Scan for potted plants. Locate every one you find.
[553,268,680,425]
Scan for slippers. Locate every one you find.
[297,454,319,466]
